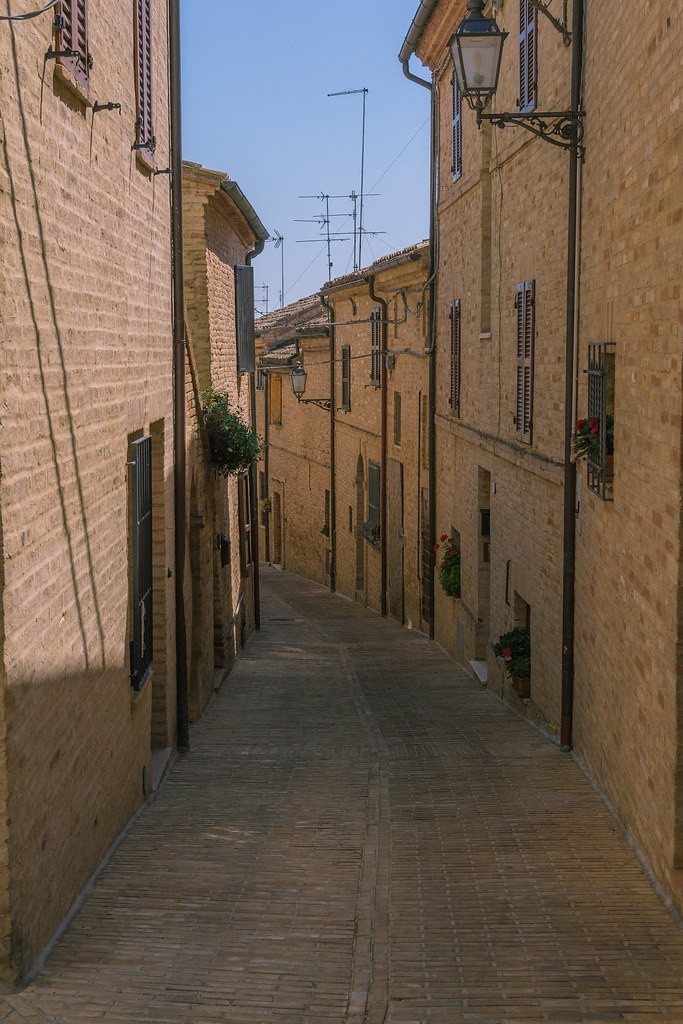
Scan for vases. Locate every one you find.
[592,450,614,477]
[450,584,461,599]
[508,671,531,699]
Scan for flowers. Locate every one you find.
[488,622,536,670]
[434,530,464,575]
[566,404,606,465]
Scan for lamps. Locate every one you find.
[443,0,586,164]
[290,365,333,413]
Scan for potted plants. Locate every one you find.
[202,386,267,476]
